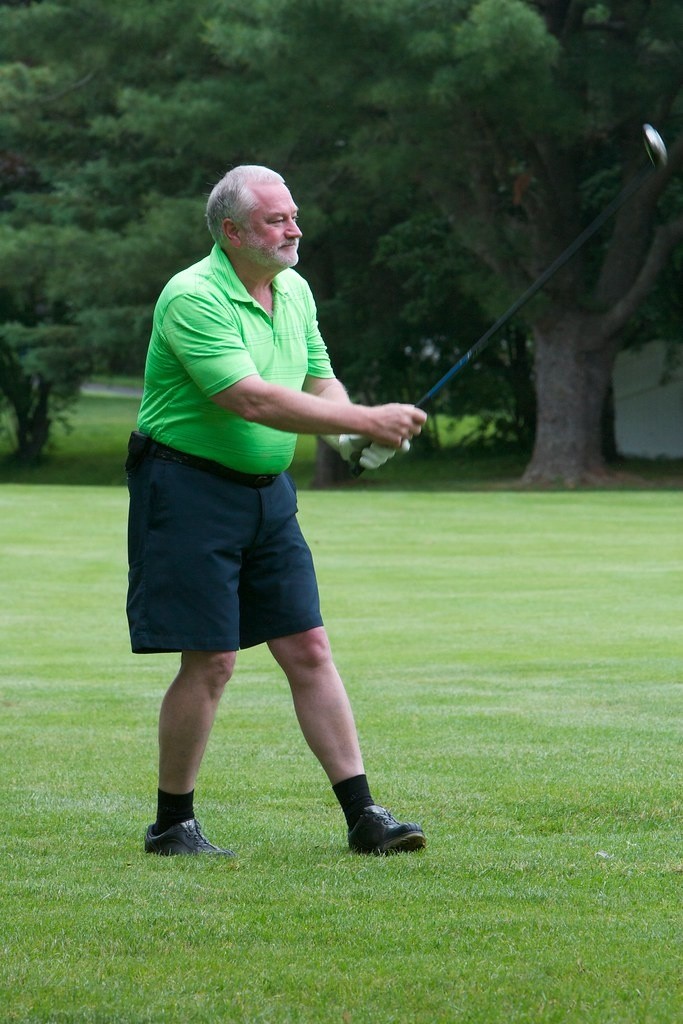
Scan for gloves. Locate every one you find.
[322,431,410,470]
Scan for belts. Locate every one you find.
[152,443,279,489]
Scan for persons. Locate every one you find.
[126,165,429,855]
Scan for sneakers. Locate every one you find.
[145,819,235,857]
[347,806,425,854]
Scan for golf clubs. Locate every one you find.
[351,122,670,481]
